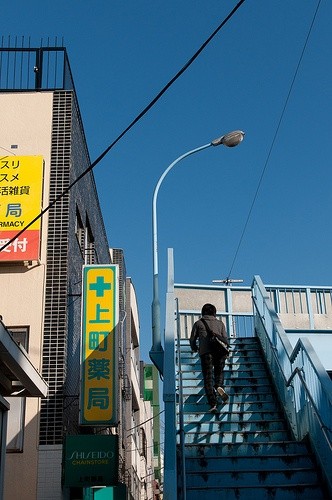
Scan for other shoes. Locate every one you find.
[208,405,218,412]
[217,387,228,401]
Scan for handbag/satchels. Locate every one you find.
[208,331,232,356]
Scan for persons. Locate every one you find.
[189,303,228,412]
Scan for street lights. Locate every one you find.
[150,130,246,499]
